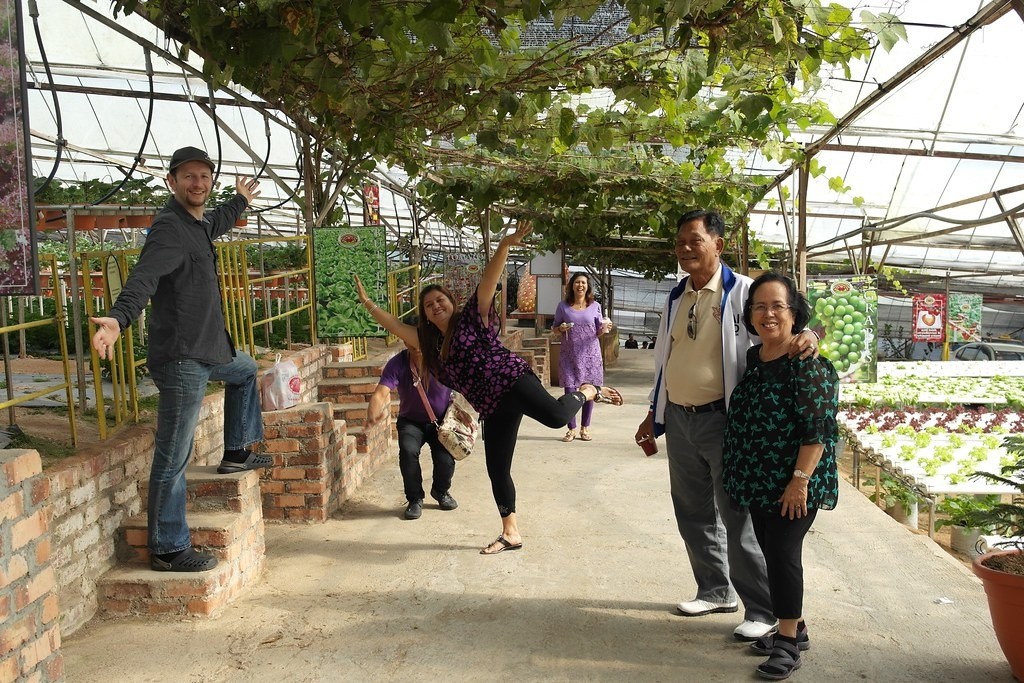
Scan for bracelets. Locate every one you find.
[368,304,377,314]
[364,299,370,305]
[558,327,562,332]
[813,331,819,340]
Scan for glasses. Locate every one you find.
[686,302,699,340]
[747,303,792,312]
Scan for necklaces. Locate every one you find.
[575,301,584,309]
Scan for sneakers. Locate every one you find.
[733,617,779,641]
[677,598,738,614]
[405,498,424,519]
[430,488,457,509]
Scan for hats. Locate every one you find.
[169,147,215,173]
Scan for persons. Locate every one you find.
[354,220,624,554]
[720,270,840,679]
[648,337,657,349]
[639,341,648,350]
[553,272,612,442]
[90,146,274,571]
[625,333,638,349]
[362,315,458,519]
[634,209,818,642]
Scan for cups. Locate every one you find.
[602,317,611,334]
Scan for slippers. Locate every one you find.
[480,535,523,553]
[579,383,623,406]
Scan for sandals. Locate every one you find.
[752,625,812,653]
[563,430,575,443]
[757,643,801,679]
[150,546,219,570]
[217,450,274,472]
[580,429,591,441]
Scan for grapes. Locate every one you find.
[813,289,866,373]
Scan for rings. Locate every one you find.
[102,344,106,347]
[808,345,814,351]
[796,506,801,509]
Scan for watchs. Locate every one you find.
[793,469,810,480]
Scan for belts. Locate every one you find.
[677,398,724,413]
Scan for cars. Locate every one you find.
[953,342,1024,361]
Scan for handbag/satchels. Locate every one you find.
[260,352,299,409]
[437,400,479,459]
